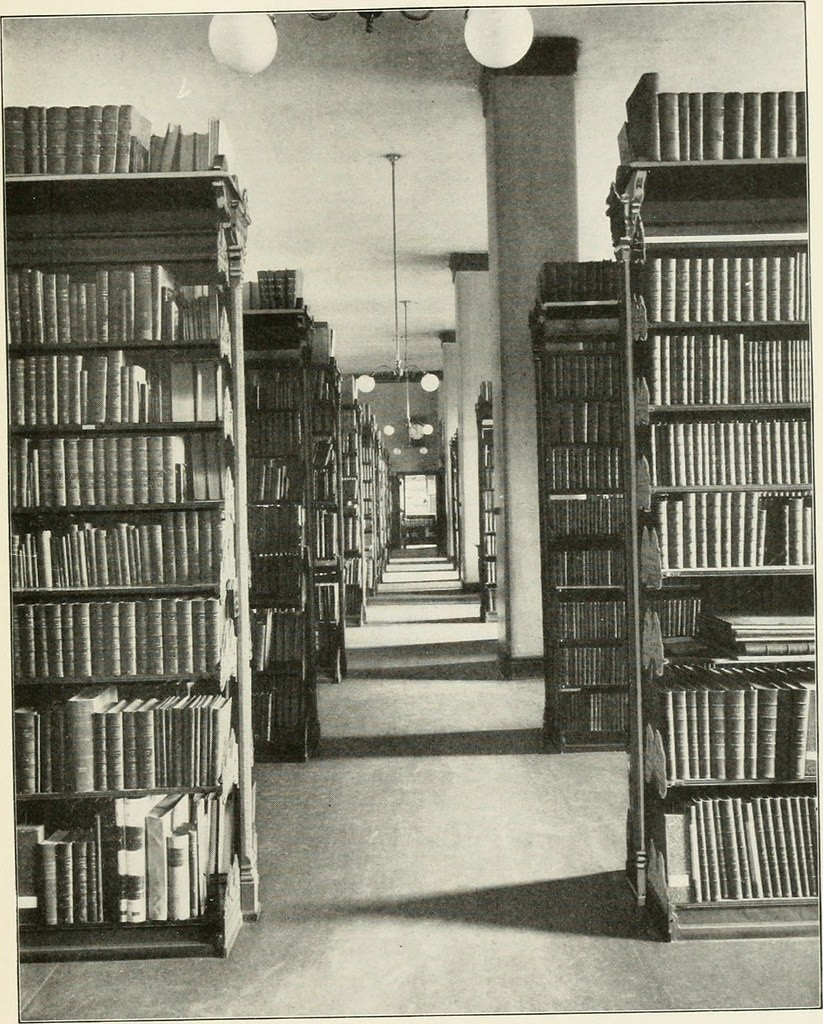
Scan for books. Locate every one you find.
[438,91,810,905]
[3,102,393,959]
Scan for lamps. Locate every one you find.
[206,7,534,73]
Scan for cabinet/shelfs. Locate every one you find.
[3,152,823,963]
